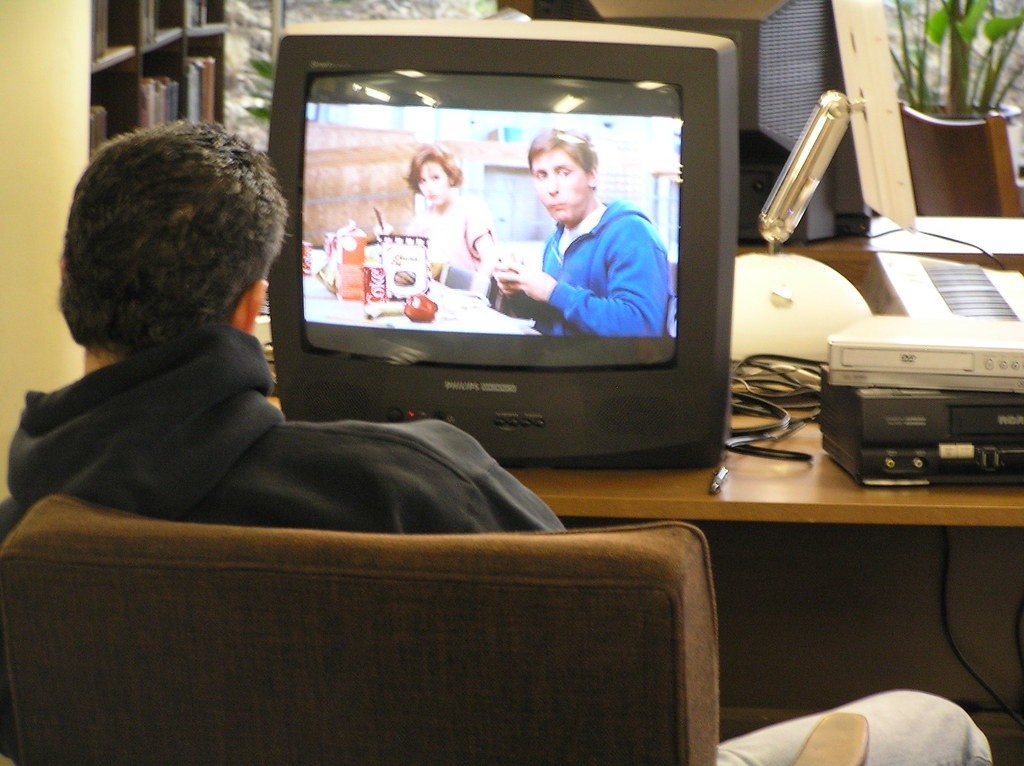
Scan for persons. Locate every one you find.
[0,120,994,766]
[491,129,669,341]
[372,139,508,299]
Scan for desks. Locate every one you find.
[266,218,1023,526]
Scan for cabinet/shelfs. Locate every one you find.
[88,1,229,157]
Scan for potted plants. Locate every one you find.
[888,0,1024,218]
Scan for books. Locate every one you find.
[186,1,210,27]
[186,54,215,126]
[138,76,180,125]
[90,105,108,155]
[143,0,158,41]
[92,0,109,62]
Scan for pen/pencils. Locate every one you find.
[709,466,729,494]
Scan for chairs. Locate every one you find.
[1,492,721,766]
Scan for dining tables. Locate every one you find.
[305,249,542,336]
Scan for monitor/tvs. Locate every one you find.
[499,0,916,250]
[269,19,739,470]
